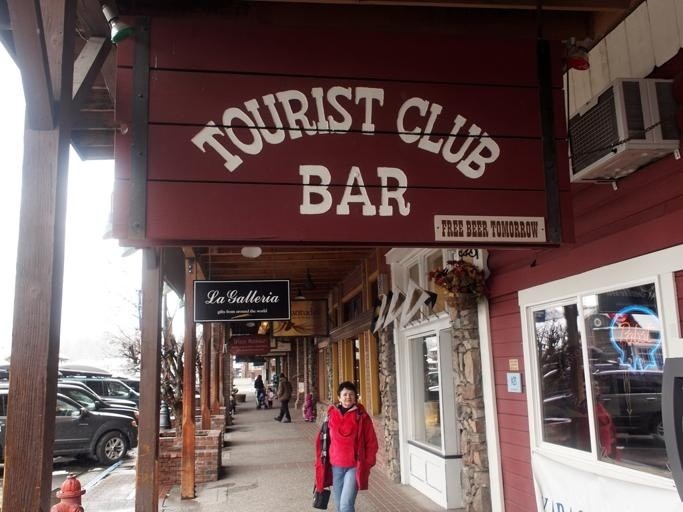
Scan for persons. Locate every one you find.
[257,389,268,409]
[266,388,274,408]
[303,394,314,424]
[274,372,292,424]
[576,379,622,462]
[314,380,379,512]
[254,375,265,403]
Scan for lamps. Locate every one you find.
[100,4,134,43]
[561,36,590,71]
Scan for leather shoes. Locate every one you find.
[283,419,291,423]
[275,418,281,421]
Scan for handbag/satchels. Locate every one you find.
[312,483,331,509]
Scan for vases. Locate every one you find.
[445,292,478,311]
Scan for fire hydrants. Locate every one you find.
[50,473,86,512]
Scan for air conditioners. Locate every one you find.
[565,77,681,191]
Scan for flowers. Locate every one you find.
[426,257,493,300]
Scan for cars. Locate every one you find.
[0,365,140,465]
[540,312,666,448]
